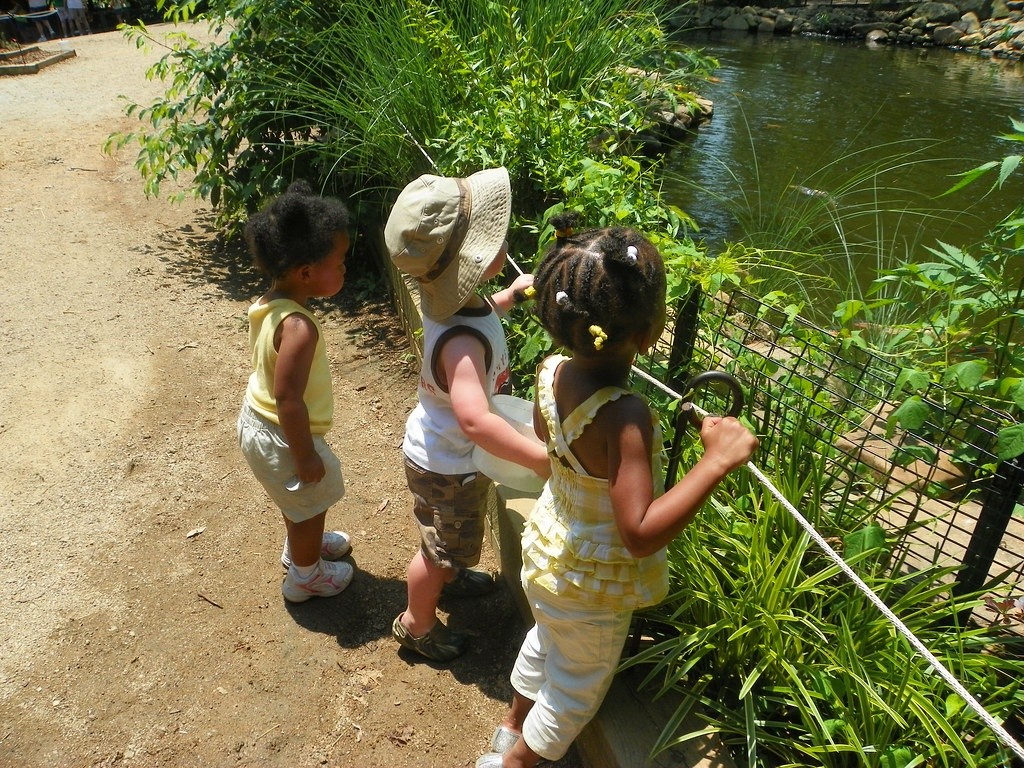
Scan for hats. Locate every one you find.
[383,166,512,322]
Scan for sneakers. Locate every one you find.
[281,558,354,602]
[280,530,351,568]
[437,569,494,612]
[391,612,469,662]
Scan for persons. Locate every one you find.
[233,188,357,604]
[473,224,761,768]
[0,0,127,44]
[381,165,553,663]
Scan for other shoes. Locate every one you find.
[491,727,521,752]
[38,37,46,42]
[50,29,54,39]
[476,752,503,768]
